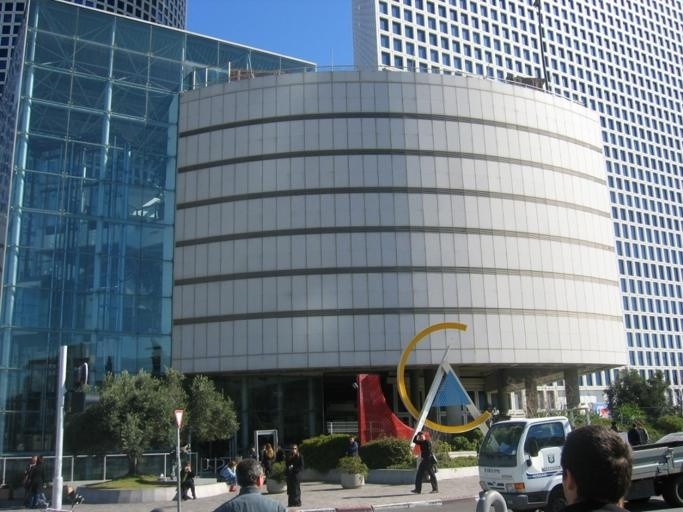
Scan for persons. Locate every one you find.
[218,441,304,506]
[343,436,358,457]
[551,424,632,512]
[26,455,51,509]
[637,422,648,443]
[21,454,38,506]
[212,458,288,511]
[409,431,438,493]
[180,460,196,500]
[628,423,641,446]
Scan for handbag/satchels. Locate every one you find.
[422,452,437,465]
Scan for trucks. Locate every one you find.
[476,415,682,512]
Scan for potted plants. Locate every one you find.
[266,461,287,493]
[338,455,369,489]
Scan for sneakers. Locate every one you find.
[288,500,302,507]
[411,489,421,494]
[429,489,439,493]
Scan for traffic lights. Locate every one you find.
[63,364,88,394]
